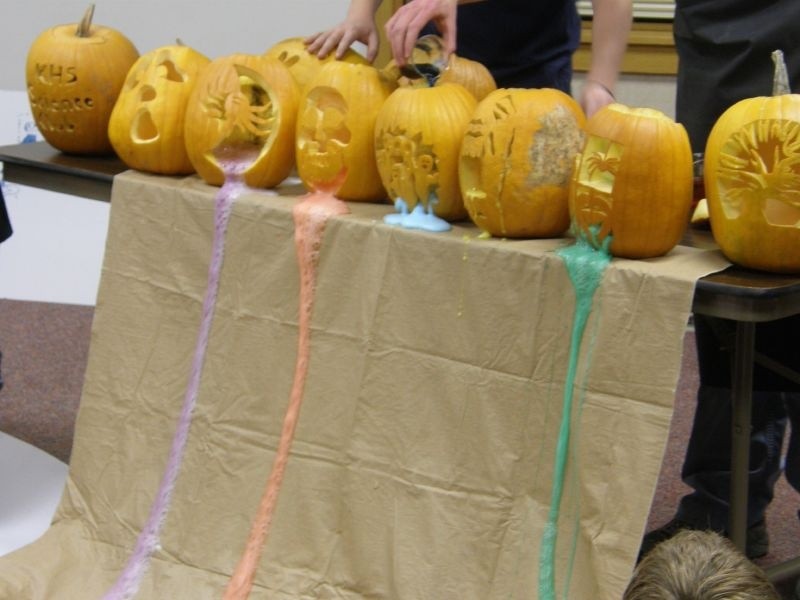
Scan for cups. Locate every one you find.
[398,36,449,80]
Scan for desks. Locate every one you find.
[0,141,799,584]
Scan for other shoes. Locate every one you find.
[638,493,769,562]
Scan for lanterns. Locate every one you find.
[25,2,799,268]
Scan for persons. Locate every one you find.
[621,527,779,600]
[387,0,800,566]
[302,0,633,115]
